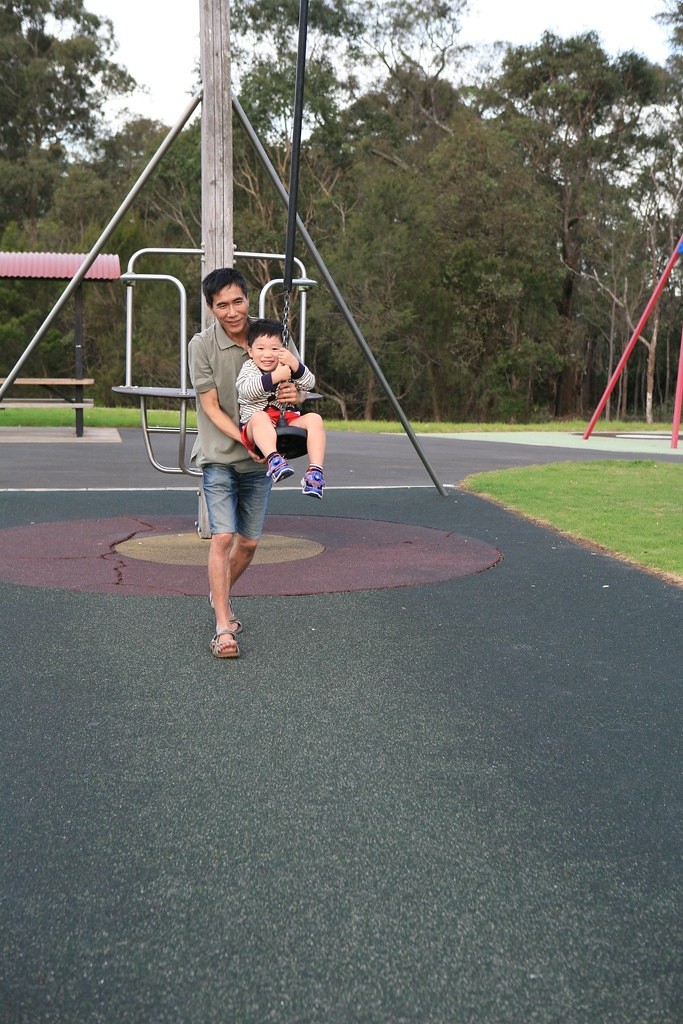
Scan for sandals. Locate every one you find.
[209,628,241,657]
[208,591,243,634]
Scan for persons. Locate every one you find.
[235,319,326,500]
[187,268,303,659]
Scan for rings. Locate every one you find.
[288,387,291,393]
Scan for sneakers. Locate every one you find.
[300,471,326,499]
[265,453,295,483]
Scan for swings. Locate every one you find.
[249,0,311,464]
